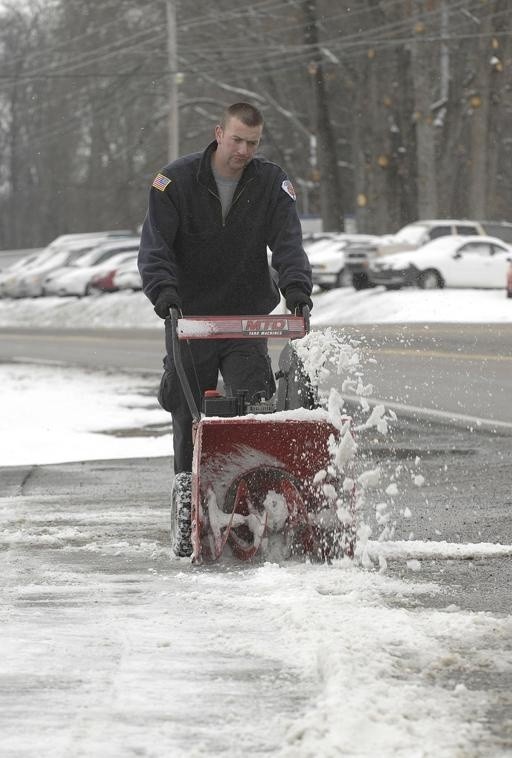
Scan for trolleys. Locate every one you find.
[164,295,361,570]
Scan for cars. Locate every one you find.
[1,229,144,300]
[263,217,512,291]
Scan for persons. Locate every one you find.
[138,103,314,475]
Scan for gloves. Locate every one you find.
[284,286,312,315]
[154,285,182,320]
